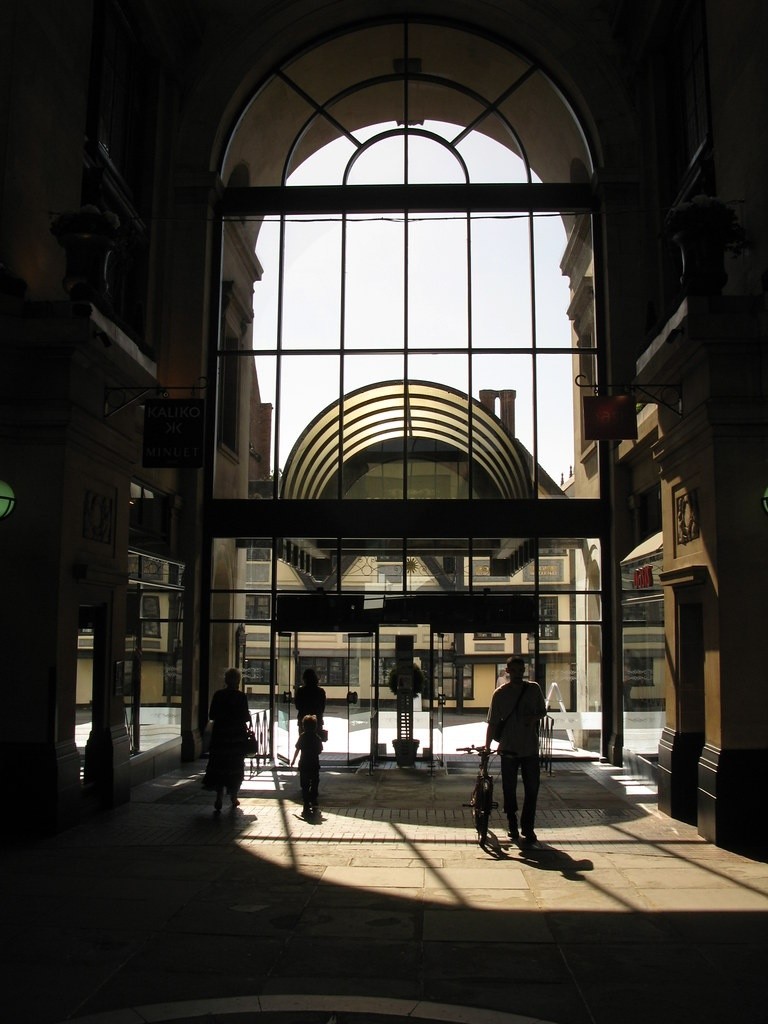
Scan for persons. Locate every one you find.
[483,656,547,842]
[202,668,251,810]
[291,715,323,817]
[294,669,326,737]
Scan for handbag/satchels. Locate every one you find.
[244,715,258,757]
[494,721,503,742]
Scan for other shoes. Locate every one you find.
[508,831,519,838]
[522,829,537,841]
[232,800,240,806]
[215,801,222,809]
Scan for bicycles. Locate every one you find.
[458,746,500,846]
